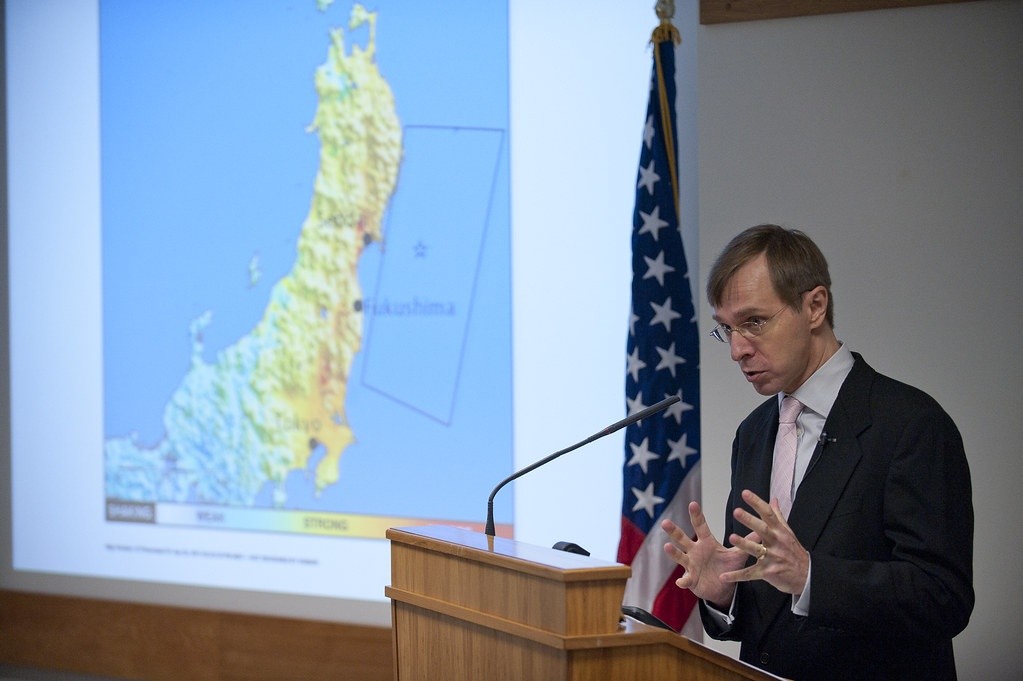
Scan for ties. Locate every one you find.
[769,397,806,523]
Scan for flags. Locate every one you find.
[616,19,705,643]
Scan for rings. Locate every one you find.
[758,546,767,560]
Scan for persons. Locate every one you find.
[661,224,975,681]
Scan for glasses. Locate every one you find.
[709,291,813,344]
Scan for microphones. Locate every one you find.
[486,395,681,536]
[820,433,828,449]
